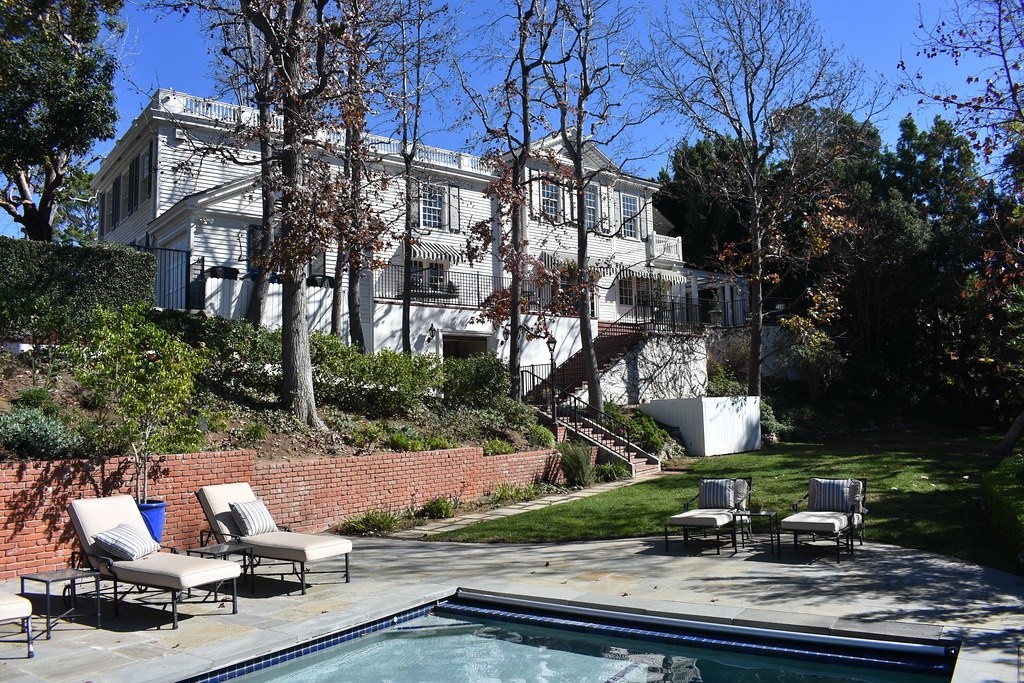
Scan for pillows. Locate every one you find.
[228,498,279,537]
[701,479,737,508]
[806,479,853,512]
[91,523,161,561]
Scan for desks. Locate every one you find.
[187,543,254,602]
[733,511,779,554]
[21,568,101,640]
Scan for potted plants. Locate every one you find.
[708,288,723,325]
[748,499,764,512]
[652,273,667,322]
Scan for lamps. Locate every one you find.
[501,327,509,346]
[427,324,436,344]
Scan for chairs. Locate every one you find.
[0,589,34,658]
[780,477,869,564]
[664,476,752,555]
[194,481,353,595]
[66,495,238,630]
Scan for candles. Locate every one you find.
[69,300,208,543]
